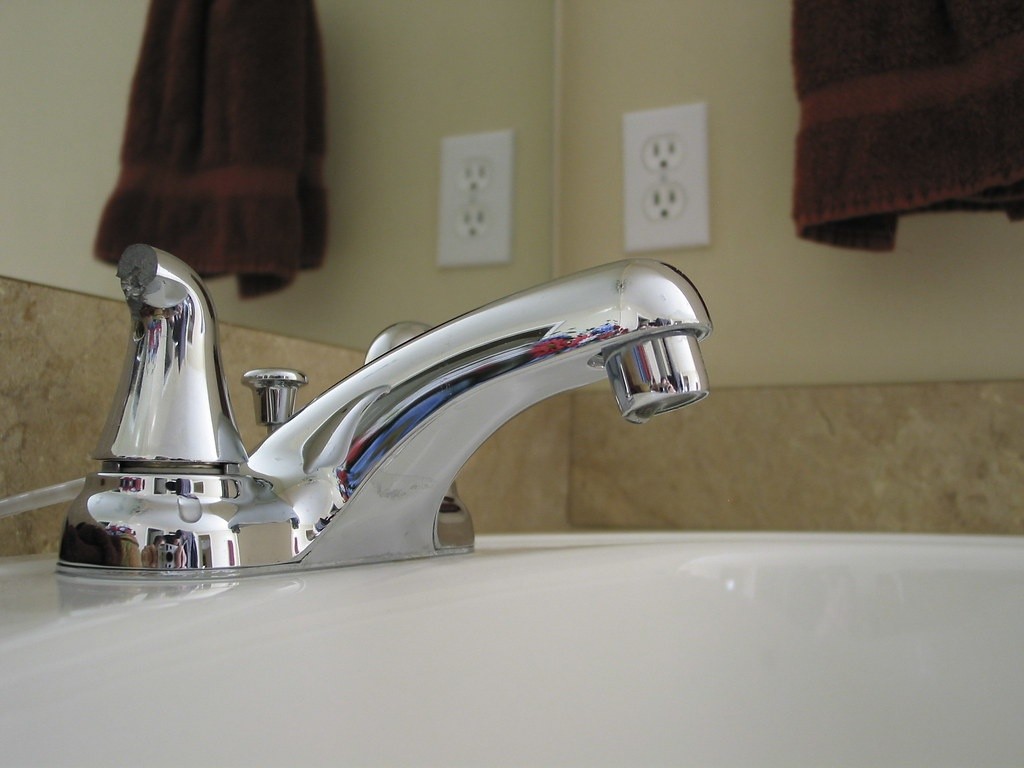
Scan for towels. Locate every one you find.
[786,0,1024,259]
[90,2,334,305]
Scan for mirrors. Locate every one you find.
[0,0,558,350]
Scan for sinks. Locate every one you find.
[0,527,1024,768]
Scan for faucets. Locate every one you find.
[51,236,715,586]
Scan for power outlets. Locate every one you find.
[619,100,712,254]
[434,128,515,270]
